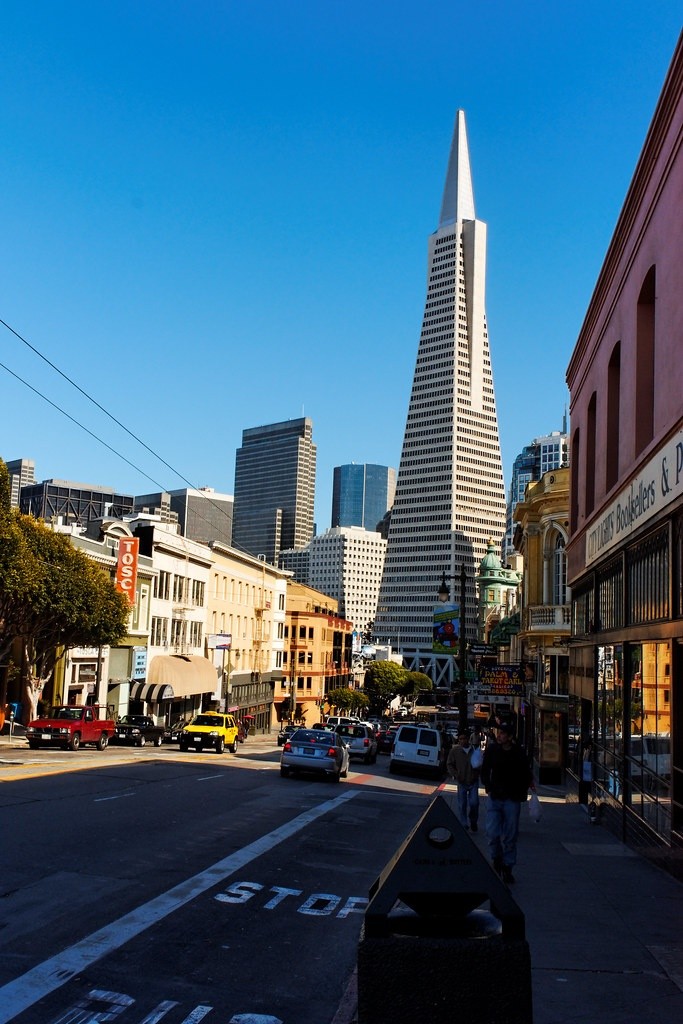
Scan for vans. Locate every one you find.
[617,736,671,792]
[388,725,443,782]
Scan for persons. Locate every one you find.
[447,730,480,832]
[606,740,620,800]
[583,743,594,762]
[436,721,450,740]
[481,722,536,884]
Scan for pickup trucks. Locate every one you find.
[25,705,117,751]
[111,715,165,747]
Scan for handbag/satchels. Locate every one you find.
[471,745,485,768]
[583,762,593,781]
[529,791,542,816]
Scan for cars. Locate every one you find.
[164,720,189,742]
[277,726,308,746]
[279,729,351,784]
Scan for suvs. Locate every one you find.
[180,711,245,754]
[311,717,399,764]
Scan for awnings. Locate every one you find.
[148,655,217,699]
[129,680,174,704]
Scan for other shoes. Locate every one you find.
[471,823,478,832]
[502,869,517,884]
[492,859,501,871]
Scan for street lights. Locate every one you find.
[288,655,312,724]
[435,563,475,745]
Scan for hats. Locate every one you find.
[497,722,513,736]
